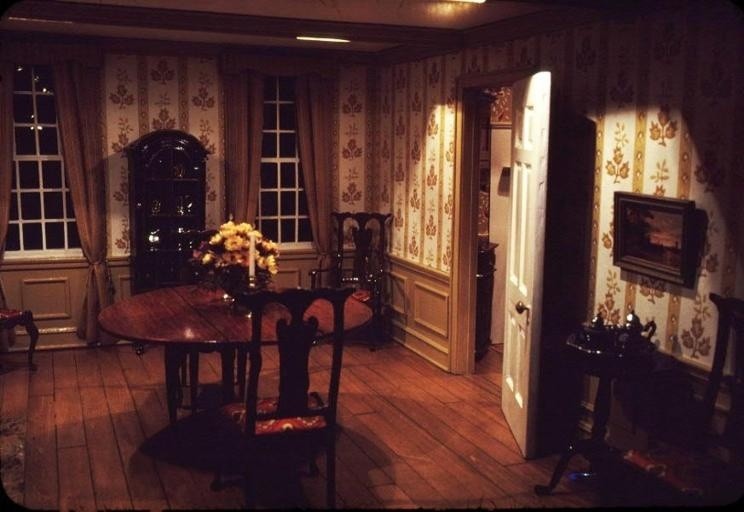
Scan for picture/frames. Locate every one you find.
[611,191,698,288]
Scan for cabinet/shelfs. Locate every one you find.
[475,237,500,363]
[122,128,211,298]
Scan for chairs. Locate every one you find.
[160,228,356,511]
[0,283,39,371]
[307,211,394,353]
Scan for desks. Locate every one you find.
[97,283,374,490]
[531,328,678,506]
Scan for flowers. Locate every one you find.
[200,218,282,277]
